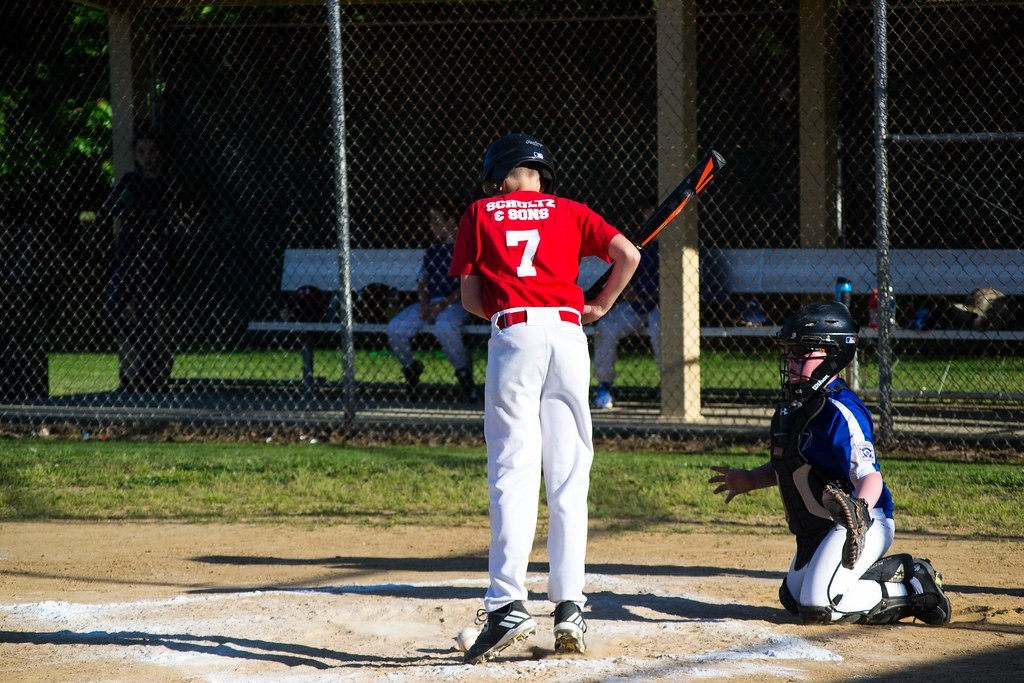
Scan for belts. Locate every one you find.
[498,310,580,325]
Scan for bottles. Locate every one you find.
[835,274,852,312]
[867,289,881,330]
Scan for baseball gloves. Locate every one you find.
[821,481,876,571]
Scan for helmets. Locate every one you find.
[775,301,859,400]
[480,133,558,196]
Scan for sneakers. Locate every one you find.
[912,558,954,624]
[551,601,588,655]
[464,600,536,665]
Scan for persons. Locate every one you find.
[447,134,643,664]
[709,301,954,626]
[593,204,668,408]
[386,197,477,403]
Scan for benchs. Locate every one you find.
[251,247,1024,383]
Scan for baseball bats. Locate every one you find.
[585,149,726,299]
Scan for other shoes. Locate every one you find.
[403,360,423,385]
[594,390,613,409]
[459,381,477,401]
